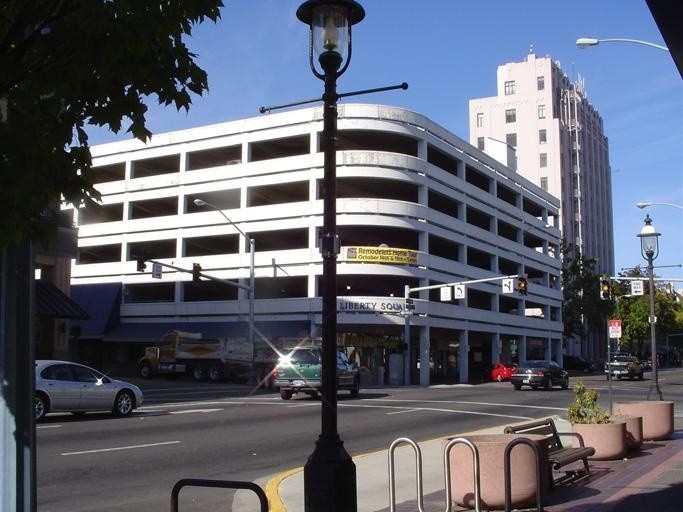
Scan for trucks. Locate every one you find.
[250,336,322,392]
[137,327,253,379]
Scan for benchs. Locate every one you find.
[504,418,595,491]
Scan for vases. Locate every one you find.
[609,415,642,450]
[441,434,548,508]
[612,401,674,441]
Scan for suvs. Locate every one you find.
[562,354,598,374]
[272,344,360,400]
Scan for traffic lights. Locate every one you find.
[137,252,147,272]
[191,263,201,285]
[599,278,611,300]
[516,273,528,295]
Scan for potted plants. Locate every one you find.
[564,379,626,461]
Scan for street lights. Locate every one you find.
[259,1,412,509]
[193,198,253,346]
[615,294,633,316]
[619,212,682,402]
[635,202,682,211]
[574,37,670,53]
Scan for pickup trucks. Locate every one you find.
[604,354,644,381]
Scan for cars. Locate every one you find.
[509,359,569,390]
[636,351,666,371]
[489,361,520,382]
[33,357,143,421]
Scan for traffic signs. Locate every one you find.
[501,278,514,294]
[454,284,466,299]
[405,298,413,304]
[405,304,415,309]
[151,263,162,279]
[629,280,643,296]
